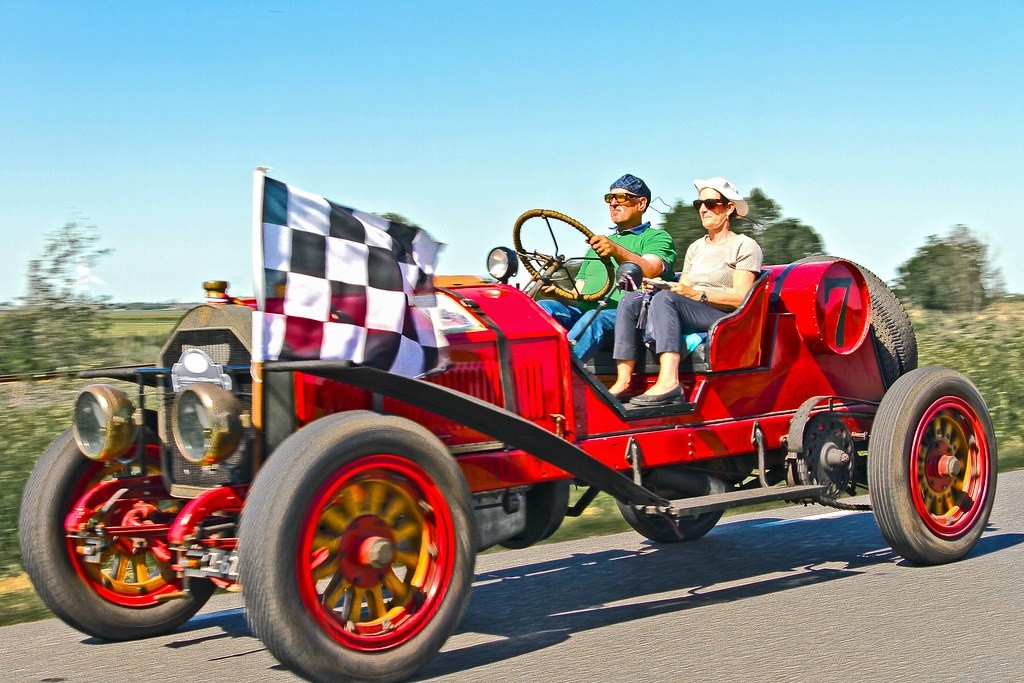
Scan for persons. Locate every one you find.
[534,173,678,365]
[608,177,764,409]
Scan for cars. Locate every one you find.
[16,207,999,683]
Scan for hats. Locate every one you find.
[693,177,749,218]
[610,174,651,208]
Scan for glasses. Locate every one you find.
[693,199,728,211]
[605,193,642,203]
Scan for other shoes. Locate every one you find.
[616,387,635,403]
[630,385,685,405]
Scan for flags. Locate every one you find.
[251,172,451,379]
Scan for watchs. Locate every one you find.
[700,291,707,303]
